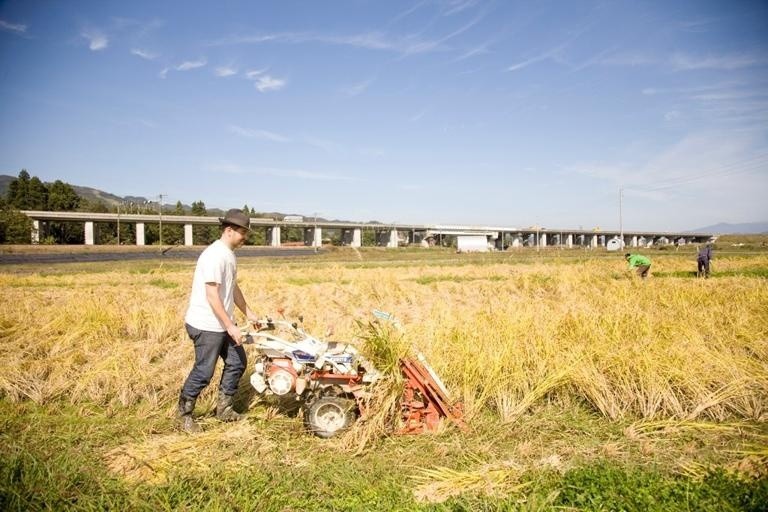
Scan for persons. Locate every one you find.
[696,241,713,279]
[624,252,653,280]
[172,207,263,437]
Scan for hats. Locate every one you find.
[218,209,253,234]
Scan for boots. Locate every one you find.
[216,388,243,421]
[176,389,202,436]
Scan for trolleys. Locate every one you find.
[232,317,465,439]
